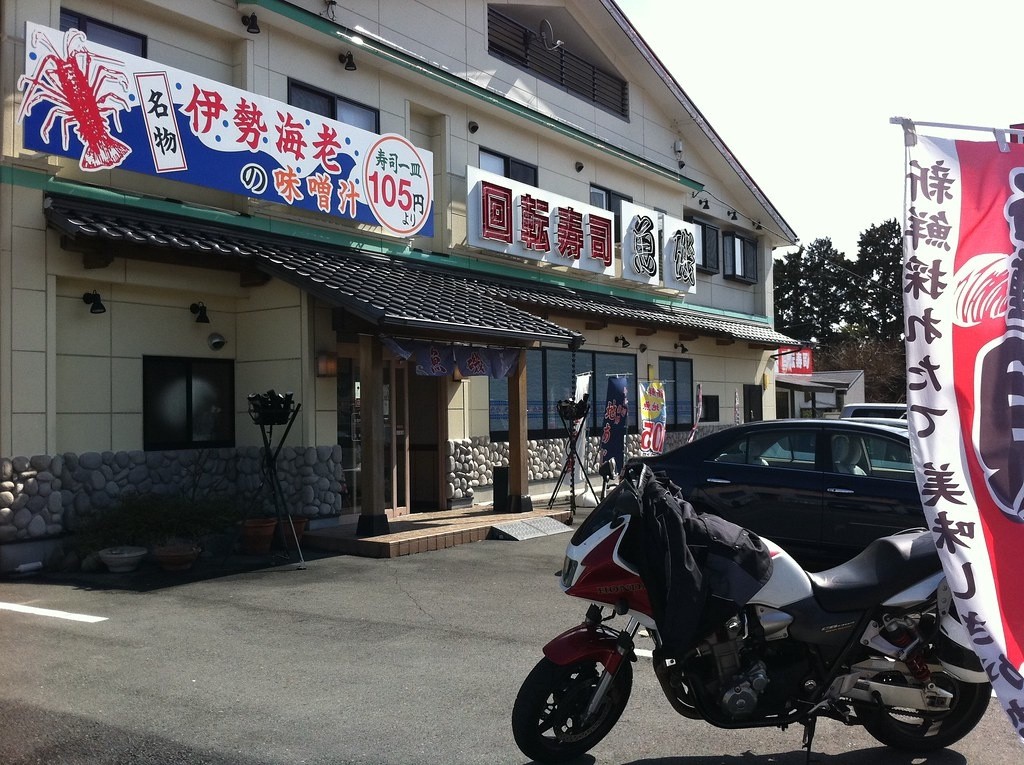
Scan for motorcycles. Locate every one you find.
[512,460,992,765]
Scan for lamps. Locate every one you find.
[727,211,738,220]
[190,301,210,324]
[615,335,630,348]
[315,350,338,378]
[241,12,260,34]
[674,342,688,354]
[452,361,468,383]
[339,51,356,70]
[82,289,106,315]
[699,200,709,209]
[752,221,761,229]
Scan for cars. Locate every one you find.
[620,419,930,573]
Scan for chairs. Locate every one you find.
[844,439,866,476]
[832,436,850,473]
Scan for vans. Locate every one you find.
[838,403,908,423]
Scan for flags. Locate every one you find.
[734,394,739,427]
[903,132,1023,741]
[639,382,668,456]
[685,384,705,442]
[560,375,591,484]
[598,377,629,476]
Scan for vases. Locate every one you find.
[152,543,201,570]
[98,545,148,573]
[270,516,309,549]
[237,518,278,544]
